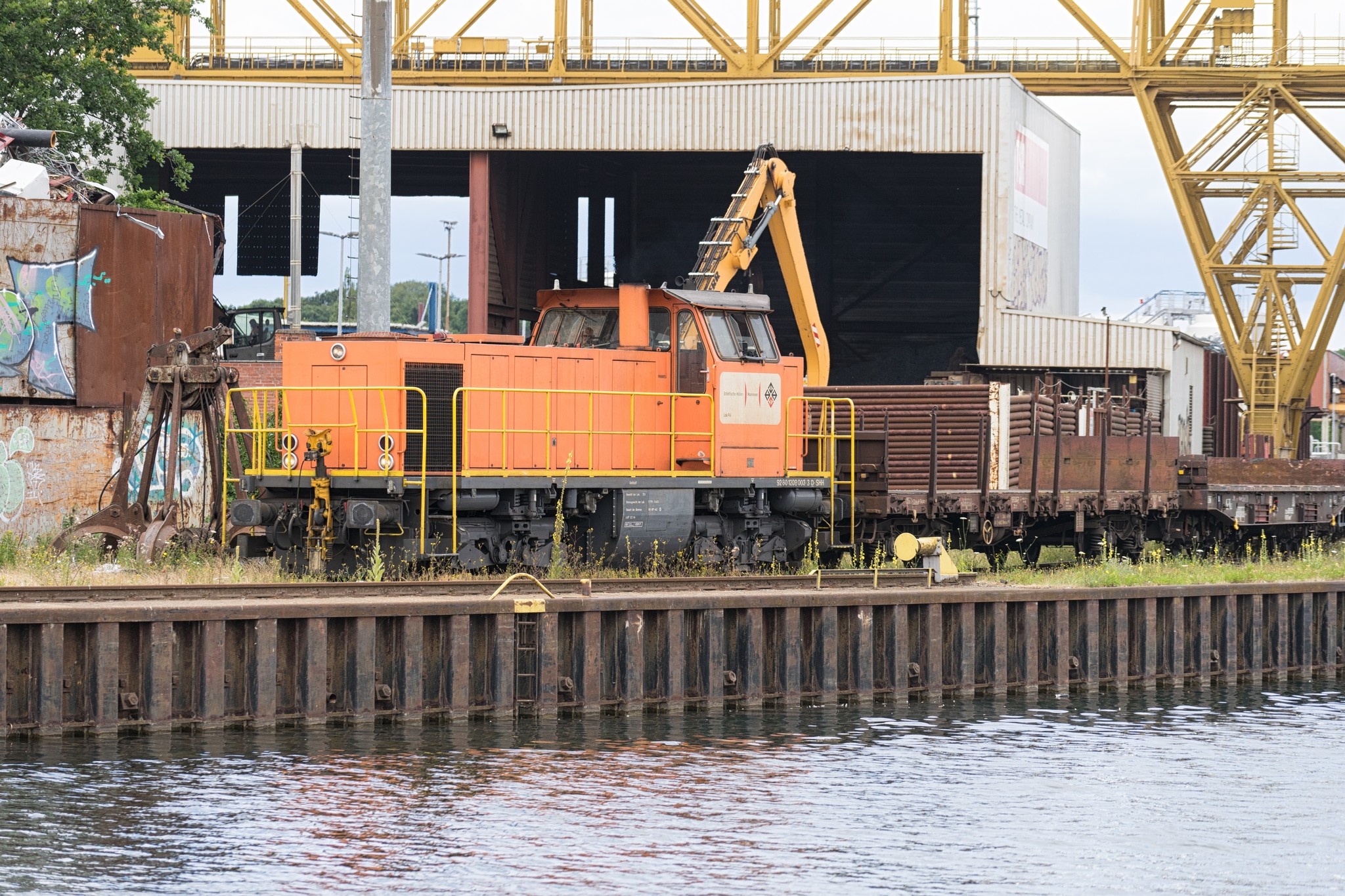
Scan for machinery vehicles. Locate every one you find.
[223,140,837,568]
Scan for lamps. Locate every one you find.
[492,123,508,138]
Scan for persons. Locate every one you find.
[649,330,654,347]
[573,326,596,348]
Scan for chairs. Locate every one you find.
[263,319,269,343]
